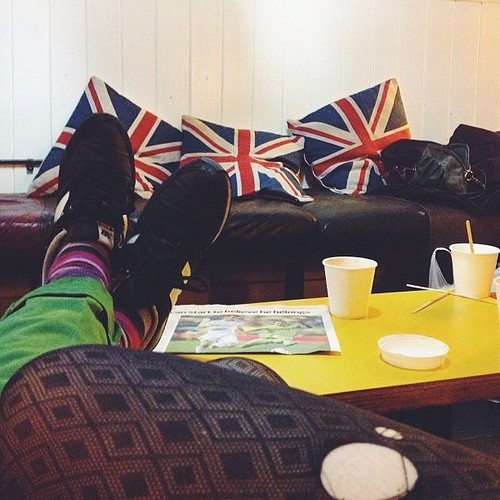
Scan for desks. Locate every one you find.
[173,288,500,414]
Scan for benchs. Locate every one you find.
[0,192,500,306]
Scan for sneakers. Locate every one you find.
[109,158,233,351]
[40,113,137,294]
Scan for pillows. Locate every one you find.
[286,78,410,195]
[181,115,315,205]
[27,76,183,200]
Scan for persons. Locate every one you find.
[1,113,231,391]
[173,315,329,352]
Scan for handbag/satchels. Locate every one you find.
[381,135,487,205]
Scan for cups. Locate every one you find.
[322,256,379,319]
[492,277,500,312]
[449,242,500,299]
